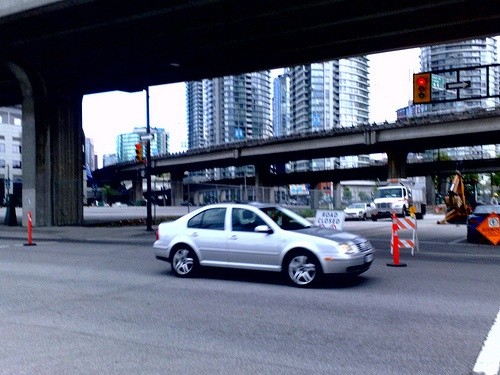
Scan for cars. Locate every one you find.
[343,201,377,222]
[152,201,376,289]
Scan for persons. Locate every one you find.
[490,192,499,205]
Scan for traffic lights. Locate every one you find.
[134,143,143,161]
[412,72,431,105]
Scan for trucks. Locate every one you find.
[373,185,426,220]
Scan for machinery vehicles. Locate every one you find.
[437,170,483,224]
